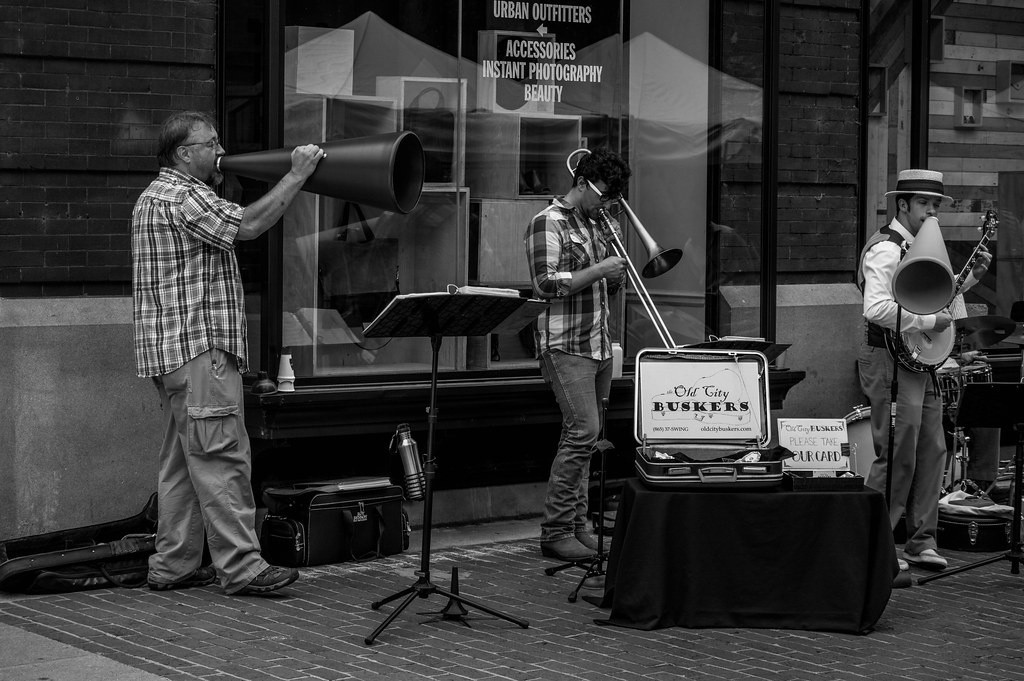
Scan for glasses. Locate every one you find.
[585,180,622,203]
[184,139,221,150]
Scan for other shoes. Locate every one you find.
[540,537,598,561]
[903,549,948,569]
[898,559,909,571]
[576,532,610,551]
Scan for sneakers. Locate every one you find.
[147,567,216,591]
[235,566,299,597]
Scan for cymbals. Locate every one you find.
[949,315,1016,359]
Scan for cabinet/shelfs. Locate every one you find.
[280,76,588,378]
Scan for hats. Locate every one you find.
[884,169,954,207]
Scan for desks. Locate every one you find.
[581,478,900,637]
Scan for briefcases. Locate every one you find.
[634,347,784,482]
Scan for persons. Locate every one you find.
[524,147,631,561]
[858,168,993,572]
[131,111,324,594]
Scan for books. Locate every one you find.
[281,307,360,347]
[675,334,765,349]
[363,284,519,334]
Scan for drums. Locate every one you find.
[844,403,965,509]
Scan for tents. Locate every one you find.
[285,12,609,120]
[516,31,763,128]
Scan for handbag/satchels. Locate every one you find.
[260,471,411,568]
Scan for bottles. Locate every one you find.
[277,347,296,393]
[610,343,623,378]
[397,423,427,501]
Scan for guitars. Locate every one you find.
[883,209,1000,374]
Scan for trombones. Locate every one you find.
[566,148,685,350]
